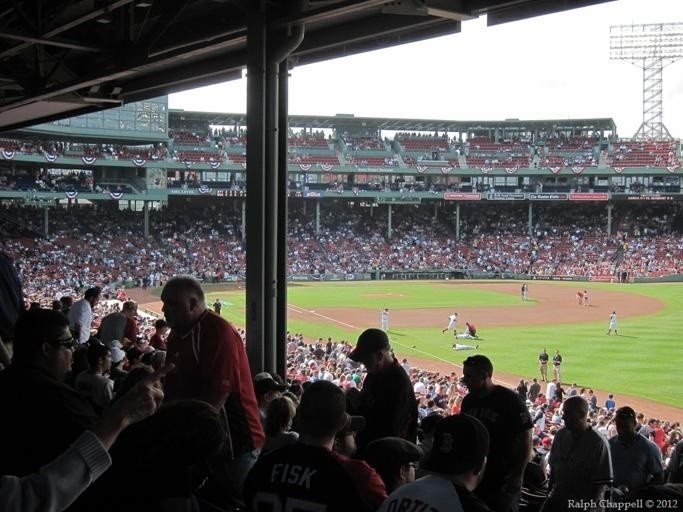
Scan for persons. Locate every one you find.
[2,126,682,512]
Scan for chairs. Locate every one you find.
[3,230,246,284]
[0,137,153,161]
[288,130,678,167]
[172,127,247,162]
[286,228,682,279]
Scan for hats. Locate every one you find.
[348,329,389,362]
[339,412,490,471]
[254,372,288,392]
